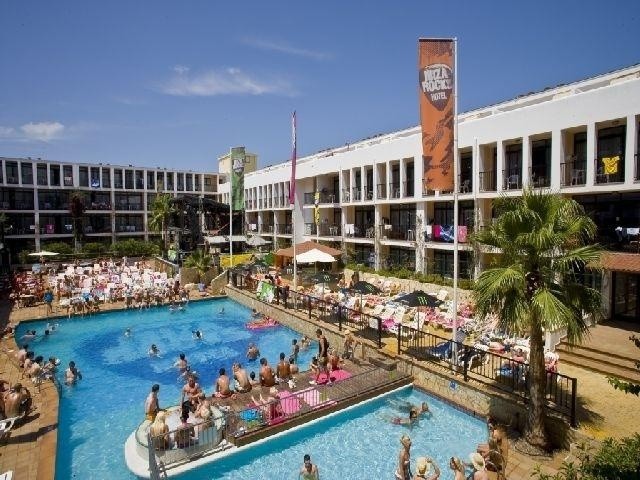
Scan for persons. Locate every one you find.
[0,252,557,480]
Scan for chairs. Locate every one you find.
[425,331,559,393]
[345,294,430,341]
[58,263,174,302]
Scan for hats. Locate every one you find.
[415,456,430,476]
[469,452,486,471]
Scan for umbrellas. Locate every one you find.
[28,250,59,256]
[292,248,336,274]
